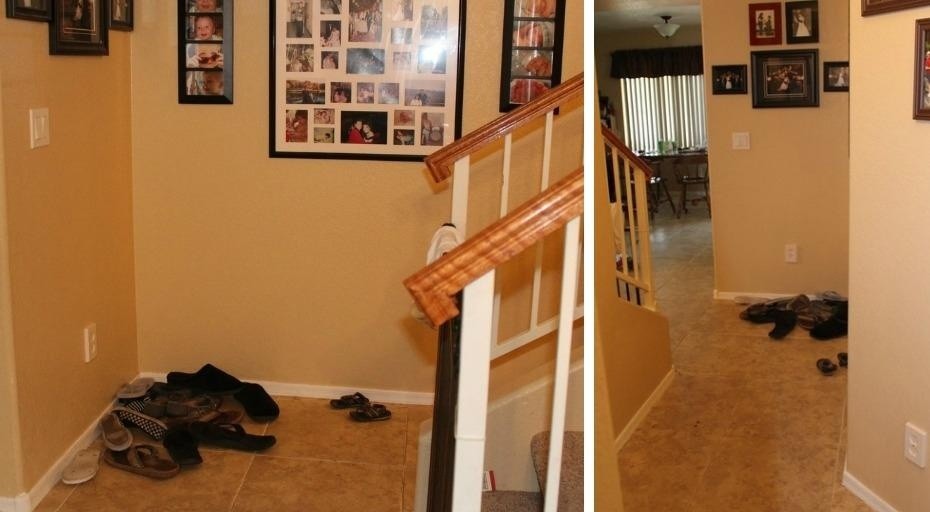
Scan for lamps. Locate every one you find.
[654,16,681,39]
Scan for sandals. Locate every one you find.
[330,393,392,421]
[817,359,833,375]
[838,353,846,367]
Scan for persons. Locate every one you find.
[511,51,552,76]
[186,43,224,67]
[716,73,745,89]
[791,8,801,36]
[516,1,556,18]
[833,68,846,87]
[511,79,549,103]
[797,14,810,36]
[764,15,773,35]
[190,17,223,42]
[287,1,445,146]
[188,70,224,95]
[757,13,765,36]
[190,1,222,13]
[512,20,555,46]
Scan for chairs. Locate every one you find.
[638,157,675,221]
[673,155,712,219]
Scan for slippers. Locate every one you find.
[102,364,280,478]
[61,448,103,486]
[736,292,848,338]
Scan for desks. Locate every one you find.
[638,149,709,207]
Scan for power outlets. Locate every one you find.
[782,242,801,267]
[904,421,927,471]
[83,321,101,366]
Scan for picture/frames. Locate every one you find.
[748,2,783,46]
[498,0,567,116]
[711,64,748,96]
[785,0,819,44]
[912,18,930,122]
[6,0,53,23]
[176,0,234,105]
[823,62,849,92]
[110,0,134,32]
[268,0,468,163]
[48,0,109,57]
[861,0,930,17]
[750,48,820,109]
